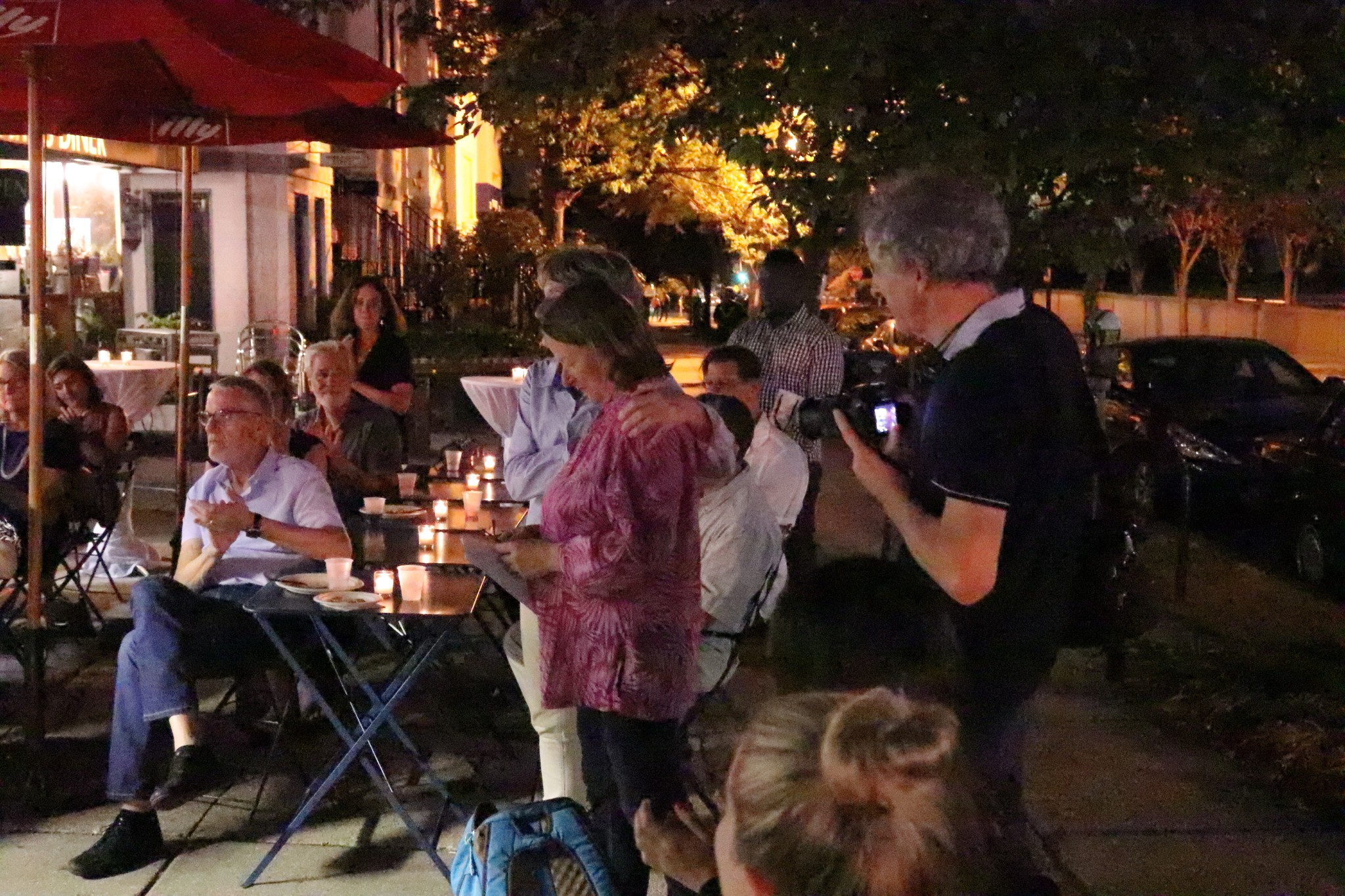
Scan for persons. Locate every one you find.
[67,373,352,880]
[0,349,127,578]
[503,248,1059,895]
[831,167,1099,810]
[242,274,415,725]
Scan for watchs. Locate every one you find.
[246,513,263,538]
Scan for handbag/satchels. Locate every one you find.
[40,590,98,637]
[1057,489,1157,688]
[0,520,20,581]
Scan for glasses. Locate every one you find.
[270,389,285,400]
[197,408,262,427]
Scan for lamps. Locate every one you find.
[118,189,152,256]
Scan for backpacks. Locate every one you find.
[450,797,623,896]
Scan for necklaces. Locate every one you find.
[0,422,30,480]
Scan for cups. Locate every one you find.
[465,474,480,490]
[364,497,386,514]
[418,524,434,549]
[512,368,523,381]
[463,491,483,517]
[432,499,448,521]
[324,557,354,590]
[445,450,462,470]
[523,369,528,377]
[396,565,427,601]
[373,570,394,599]
[397,473,416,498]
[121,351,133,366]
[484,457,496,471]
[98,350,110,366]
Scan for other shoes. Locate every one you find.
[68,820,167,879]
[150,749,231,812]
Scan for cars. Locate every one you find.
[1076,334,1344,601]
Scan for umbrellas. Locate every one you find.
[0,105,456,526]
[0,0,405,798]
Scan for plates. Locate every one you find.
[313,591,384,610]
[275,573,364,594]
[359,504,427,518]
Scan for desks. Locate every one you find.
[307,529,518,575]
[398,473,539,506]
[219,562,489,893]
[436,446,539,487]
[355,497,534,536]
[111,312,228,409]
[73,352,179,432]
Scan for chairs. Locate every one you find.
[230,318,318,411]
[785,455,841,590]
[198,656,308,826]
[0,430,147,671]
[703,535,778,746]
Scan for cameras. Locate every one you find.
[797,383,911,441]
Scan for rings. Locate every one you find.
[210,520,213,525]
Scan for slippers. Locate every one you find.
[254,709,288,733]
[298,699,330,725]
